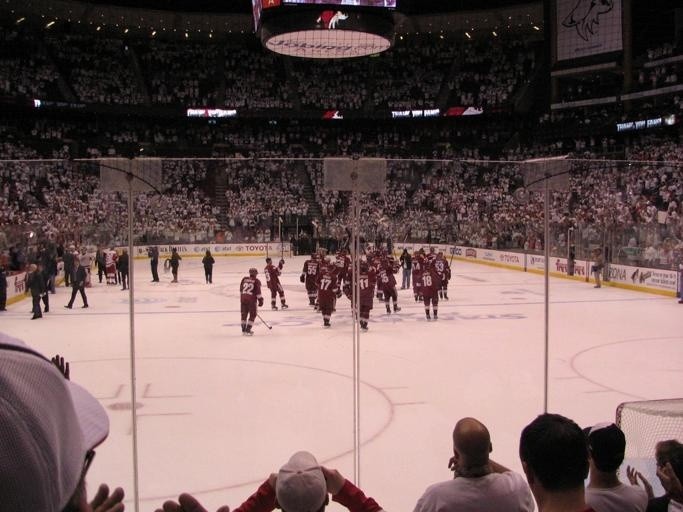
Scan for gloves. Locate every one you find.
[279,259,285,267]
[300,274,305,283]
[257,296,264,307]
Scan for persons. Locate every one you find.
[202,250,215,284]
[169,247,182,282]
[626,440,683,512]
[24,246,130,319]
[240,268,264,331]
[592,248,605,288]
[582,422,649,512]
[2,268,8,311]
[0,334,230,511]
[264,258,289,310]
[52,355,69,379]
[0,42,683,270]
[519,413,596,512]
[656,462,683,512]
[233,451,383,512]
[300,248,451,330]
[412,417,535,512]
[148,246,159,282]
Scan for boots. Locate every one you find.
[439,293,443,298]
[394,304,401,311]
[360,320,368,330]
[246,324,253,333]
[241,324,246,332]
[443,292,448,299]
[385,305,391,312]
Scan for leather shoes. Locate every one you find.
[63,305,72,308]
[80,305,89,308]
[31,314,42,319]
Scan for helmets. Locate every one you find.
[310,247,443,274]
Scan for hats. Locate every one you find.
[275,450,327,511]
[581,422,625,460]
[0,332,111,512]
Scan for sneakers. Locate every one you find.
[324,322,330,326]
